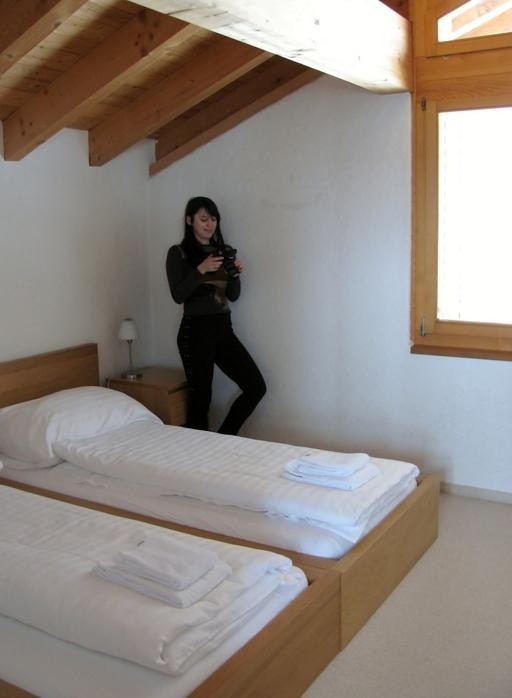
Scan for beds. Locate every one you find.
[0,479,341,698]
[0,343,440,650]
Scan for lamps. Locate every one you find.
[118,318,143,380]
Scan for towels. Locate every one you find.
[117,536,217,590]
[92,534,232,609]
[279,447,380,492]
[296,450,369,476]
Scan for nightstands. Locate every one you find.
[105,366,188,426]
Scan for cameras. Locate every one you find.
[214,247,240,279]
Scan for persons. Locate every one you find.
[165,196,266,436]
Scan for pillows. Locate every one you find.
[0,384,165,468]
